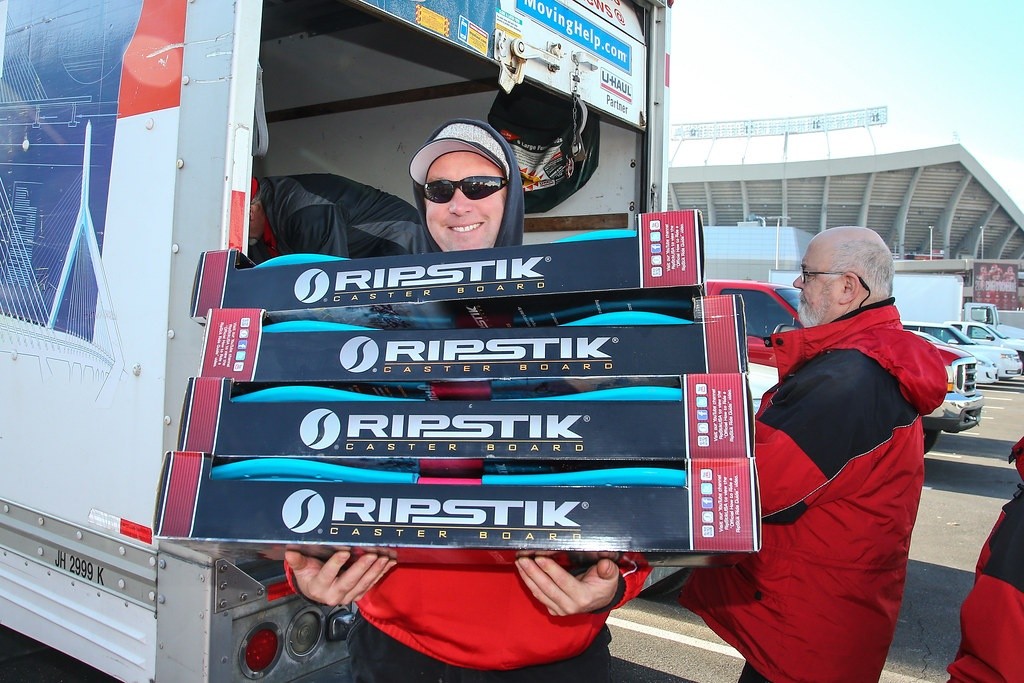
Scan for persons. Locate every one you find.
[283,118,651,683]
[250,172,429,258]
[947,436,1024,683]
[682,226,948,683]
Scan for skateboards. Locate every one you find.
[207,228,697,485]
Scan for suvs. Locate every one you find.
[702,278,985,456]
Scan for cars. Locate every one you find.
[898,321,1024,385]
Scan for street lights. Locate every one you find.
[979,225,985,259]
[766,216,792,270]
[928,225,934,260]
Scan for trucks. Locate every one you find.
[767,271,1023,340]
[0,0,672,683]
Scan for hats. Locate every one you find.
[408,122,511,187]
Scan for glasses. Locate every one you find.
[422,175,508,204]
[799,267,845,283]
